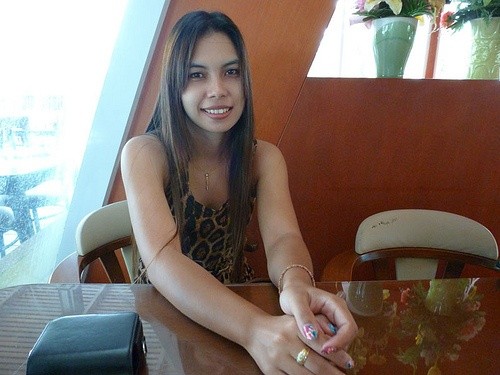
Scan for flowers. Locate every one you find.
[441,12,455,27]
[349,0,445,28]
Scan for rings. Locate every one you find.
[297,347,310,367]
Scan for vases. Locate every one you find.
[370,17,418,79]
[466,17,500,80]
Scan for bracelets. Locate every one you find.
[278,264,315,295]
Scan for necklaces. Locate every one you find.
[193,149,230,191]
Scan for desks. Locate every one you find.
[0,277,500,375]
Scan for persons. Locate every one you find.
[120,10,358,375]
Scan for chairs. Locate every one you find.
[76,200,140,284]
[350,209,500,281]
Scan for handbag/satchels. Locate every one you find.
[26,312,148,375]
